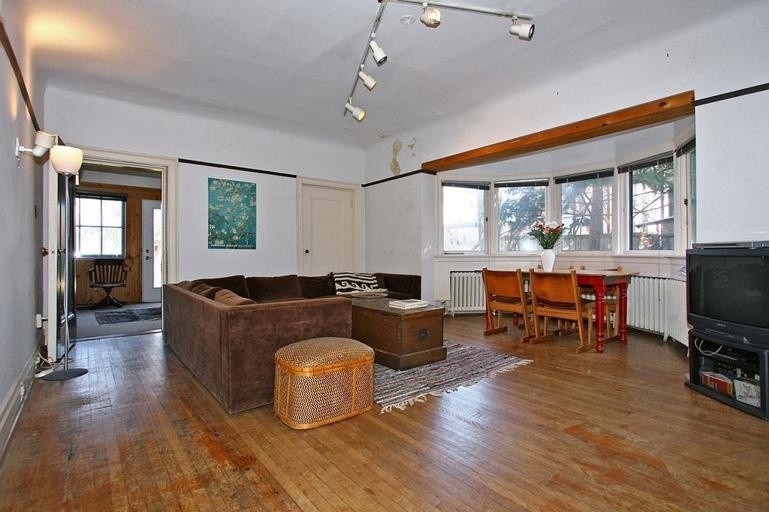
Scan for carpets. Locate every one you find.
[372,338,534,414]
[95,306,163,326]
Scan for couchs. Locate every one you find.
[163,272,422,415]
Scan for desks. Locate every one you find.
[482,270,640,353]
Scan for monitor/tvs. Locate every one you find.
[686,248,769,349]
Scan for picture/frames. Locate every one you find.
[208,177,256,249]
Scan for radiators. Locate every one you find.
[450,273,689,357]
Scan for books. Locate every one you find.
[389,299,428,309]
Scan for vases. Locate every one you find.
[541,249,555,272]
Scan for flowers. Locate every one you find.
[527,220,568,249]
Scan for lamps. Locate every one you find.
[359,63,376,90]
[421,6,441,27]
[345,96,365,120]
[15,129,57,166]
[42,145,84,382]
[370,32,388,64]
[511,16,535,41]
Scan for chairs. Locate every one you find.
[559,266,623,341]
[529,267,595,352]
[88,259,129,308]
[483,267,540,342]
[518,264,574,335]
